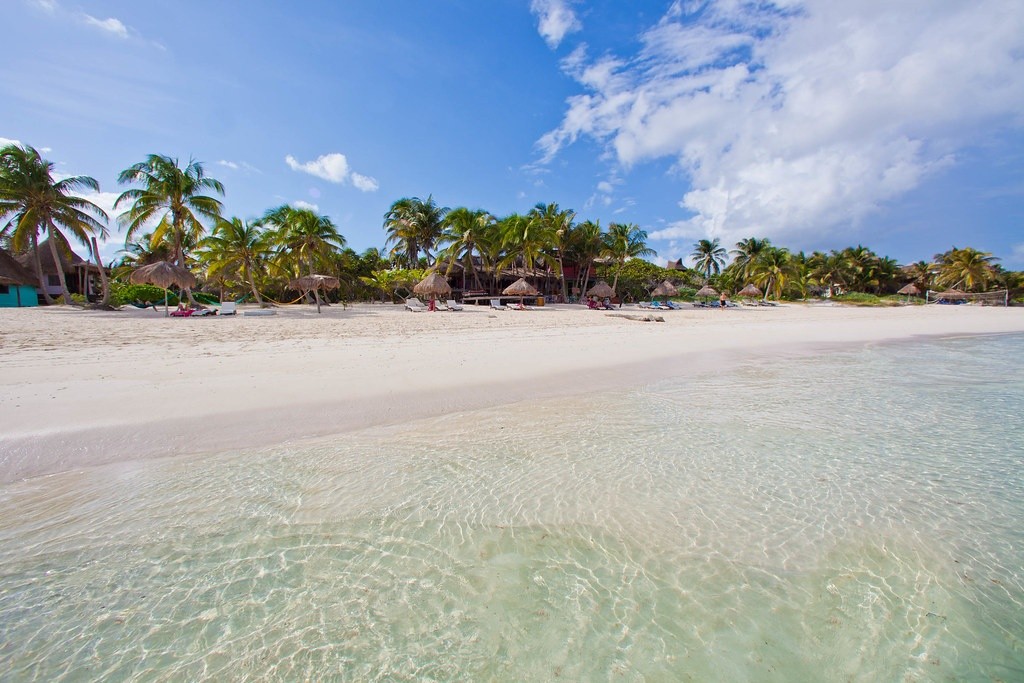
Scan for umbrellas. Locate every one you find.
[289,274,339,314]
[738,284,762,301]
[651,280,678,303]
[413,273,452,311]
[830,283,841,287]
[587,280,616,301]
[898,283,920,301]
[131,261,195,317]
[937,289,964,301]
[695,284,719,306]
[501,278,537,309]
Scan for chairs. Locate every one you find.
[171,302,235,317]
[587,302,619,310]
[742,298,778,306]
[405,298,462,312]
[692,301,736,307]
[490,300,525,310]
[639,302,683,310]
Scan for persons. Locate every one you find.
[429,297,449,312]
[900,298,905,307]
[650,299,673,307]
[587,296,613,310]
[342,296,348,310]
[817,284,845,298]
[274,294,298,304]
[720,292,727,310]
[138,299,206,312]
[516,302,526,310]
